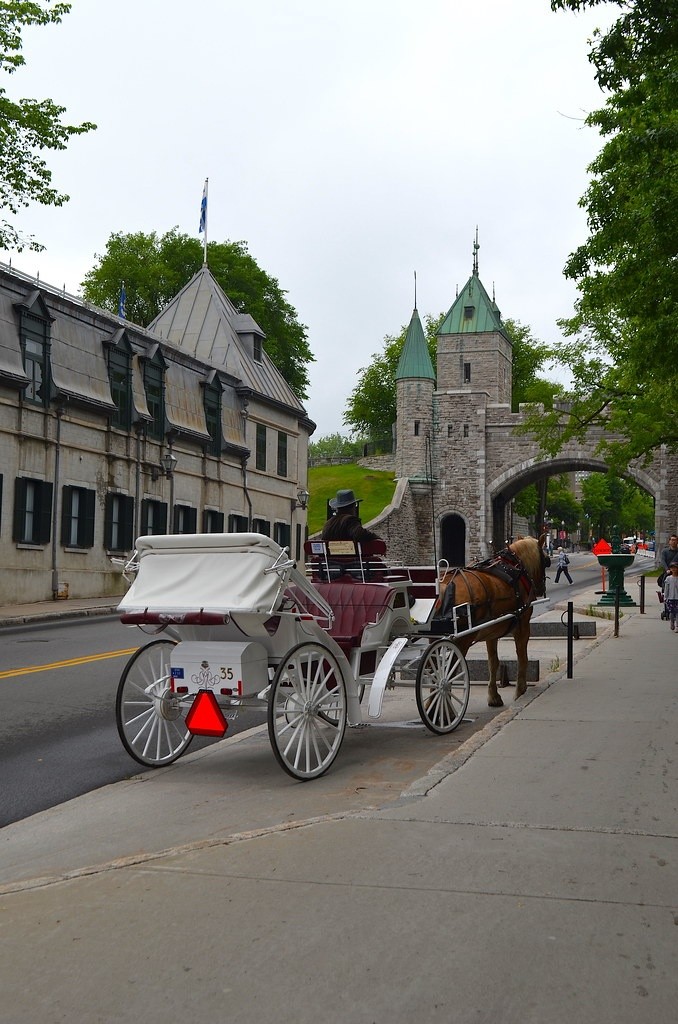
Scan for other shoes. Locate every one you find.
[671,621,675,630]
[675,627,678,633]
[568,582,574,585]
[552,582,558,584]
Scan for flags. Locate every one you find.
[119,289,126,319]
[199,189,207,234]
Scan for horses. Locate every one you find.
[424,532,552,725]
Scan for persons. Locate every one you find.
[661,533,678,633]
[552,547,575,586]
[321,489,416,608]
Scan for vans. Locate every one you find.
[622,538,636,550]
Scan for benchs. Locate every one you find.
[284,583,396,649]
[304,539,412,588]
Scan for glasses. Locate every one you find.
[353,502,358,507]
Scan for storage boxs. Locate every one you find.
[170,641,268,696]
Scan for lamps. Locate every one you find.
[152,454,177,480]
[291,490,310,510]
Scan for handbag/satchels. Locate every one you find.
[657,571,666,586]
[565,558,570,564]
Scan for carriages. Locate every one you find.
[112,527,554,782]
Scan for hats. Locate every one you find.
[669,561,678,568]
[329,490,363,510]
